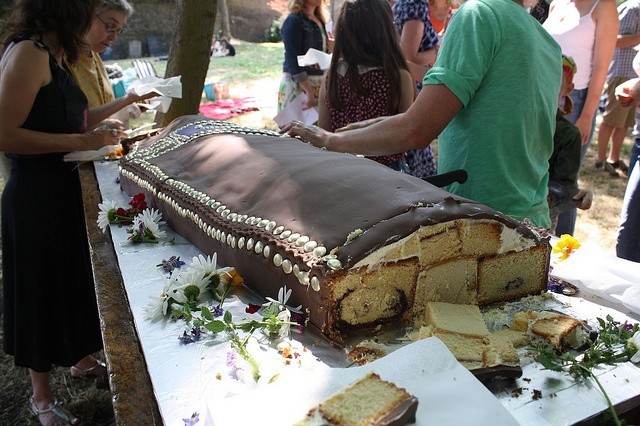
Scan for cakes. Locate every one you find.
[292,370,420,425]
[513,309,583,354]
[415,301,523,381]
[117,113,553,348]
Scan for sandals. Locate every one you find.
[70,357,107,377]
[28,393,79,426]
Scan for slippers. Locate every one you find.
[595,160,620,176]
[611,158,629,176]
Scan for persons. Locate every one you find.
[0,0,125,425]
[389,0,439,179]
[276,0,565,237]
[542,0,619,239]
[276,0,330,133]
[316,0,439,181]
[429,0,451,35]
[614,48,640,262]
[512,0,549,24]
[544,52,592,239]
[209,37,221,58]
[595,1,640,172]
[64,1,161,127]
[219,38,235,57]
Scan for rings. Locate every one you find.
[112,129,117,135]
[295,123,300,126]
[298,120,301,123]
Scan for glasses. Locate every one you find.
[95,13,127,35]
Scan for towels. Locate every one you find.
[64,144,122,163]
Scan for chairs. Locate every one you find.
[131,57,158,80]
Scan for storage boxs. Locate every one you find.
[204,81,216,102]
[111,78,126,99]
